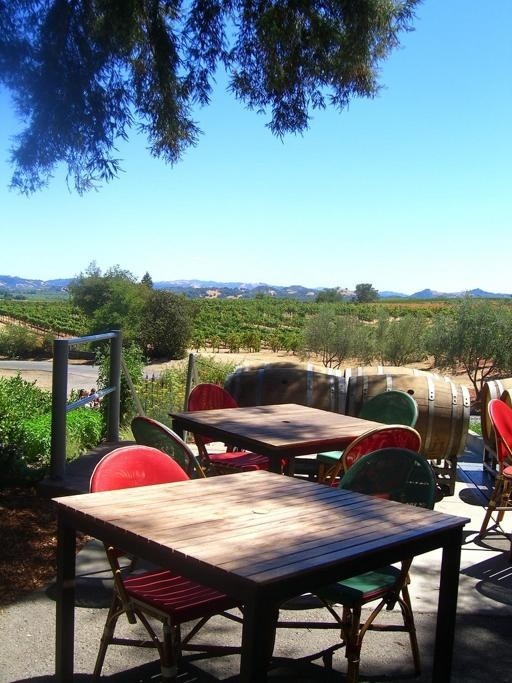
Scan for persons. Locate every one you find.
[78,386,101,409]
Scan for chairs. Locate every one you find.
[293,446,428,676]
[129,413,223,480]
[90,444,262,683]
[324,421,424,500]
[184,381,275,473]
[313,389,422,477]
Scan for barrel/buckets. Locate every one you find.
[221,360,345,414]
[481,377,511,439]
[347,365,471,460]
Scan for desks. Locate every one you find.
[168,400,398,473]
[50,470,471,683]
